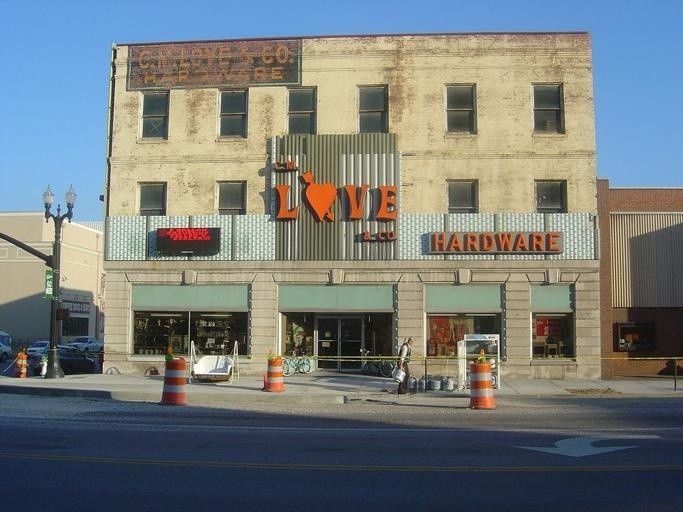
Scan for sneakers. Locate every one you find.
[396,389,409,394]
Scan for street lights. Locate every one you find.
[1,183,76,379]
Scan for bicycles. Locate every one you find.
[360,348,397,377]
[281,352,316,377]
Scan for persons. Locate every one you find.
[396,336,417,396]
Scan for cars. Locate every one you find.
[0,330,12,363]
[27,335,104,376]
